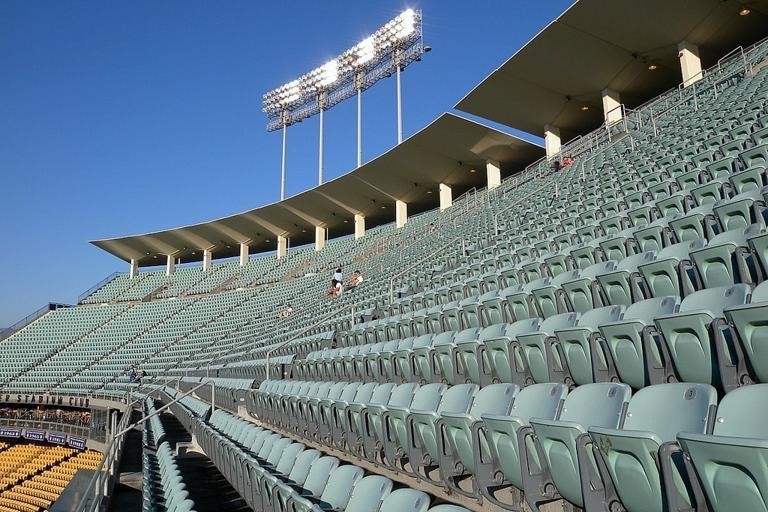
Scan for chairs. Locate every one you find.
[323,41,768,510]
[2,243,328,509]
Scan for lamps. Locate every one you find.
[294,224,298,228]
[265,238,270,242]
[257,233,260,236]
[220,239,224,242]
[301,227,307,232]
[302,60,325,99]
[262,78,305,117]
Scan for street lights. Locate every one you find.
[261,8,432,200]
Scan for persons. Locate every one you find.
[561,157,572,166]
[332,269,343,285]
[286,304,292,315]
[129,365,137,381]
[352,271,363,286]
[329,279,343,298]
[131,371,146,383]
